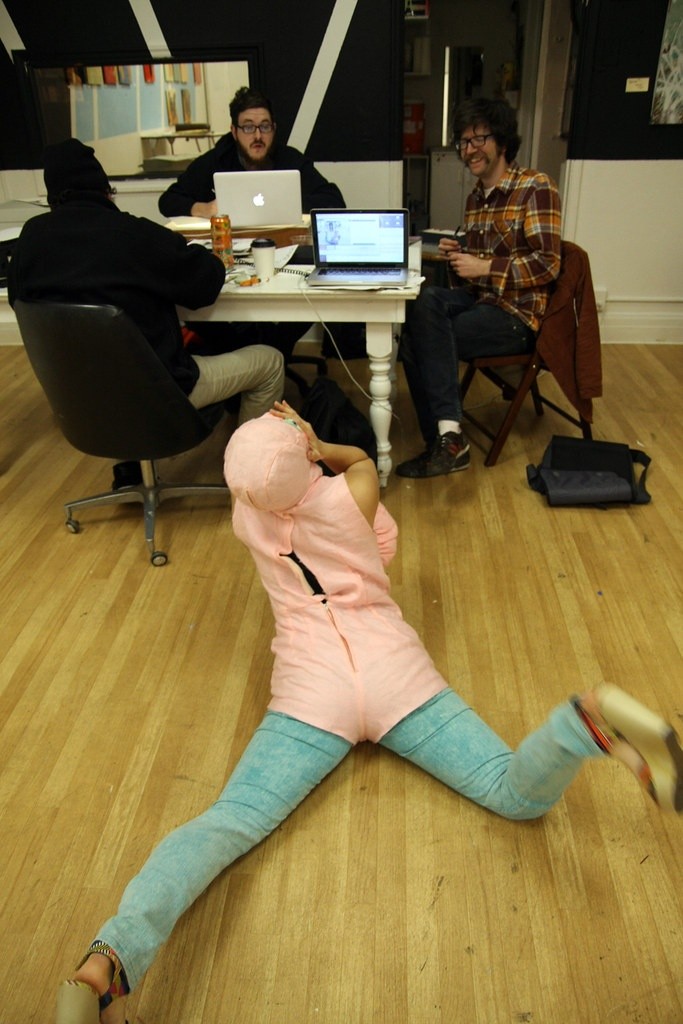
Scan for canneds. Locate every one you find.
[210,214,234,271]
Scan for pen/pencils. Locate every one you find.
[445,225,462,254]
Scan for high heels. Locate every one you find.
[575,681,683,814]
[53,941,128,1024]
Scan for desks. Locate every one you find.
[176,234,422,488]
[141,134,221,152]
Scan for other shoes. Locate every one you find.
[113,461,142,489]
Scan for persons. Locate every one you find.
[324,222,340,245]
[7,138,284,488]
[395,97,562,478]
[157,86,347,356]
[454,40,519,145]
[55,400,683,1024]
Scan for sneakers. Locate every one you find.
[397,430,470,477]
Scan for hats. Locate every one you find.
[45,137,110,204]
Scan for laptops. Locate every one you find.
[213,170,310,226]
[307,208,410,286]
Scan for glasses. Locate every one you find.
[454,133,497,151]
[235,123,273,134]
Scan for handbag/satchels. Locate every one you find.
[299,378,379,476]
[527,434,637,508]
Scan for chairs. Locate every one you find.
[461,238,591,467]
[14,299,229,564]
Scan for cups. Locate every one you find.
[250,238,276,280]
[291,235,313,245]
